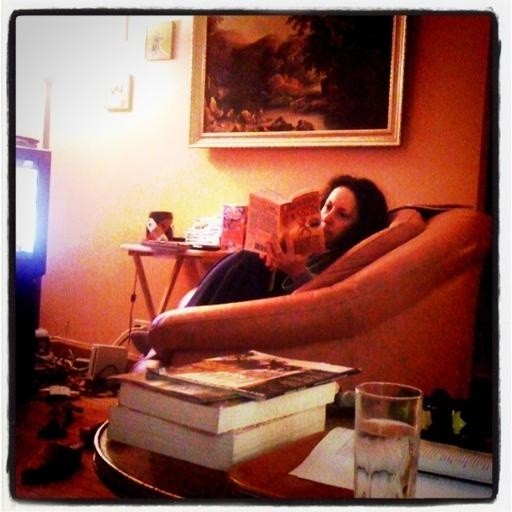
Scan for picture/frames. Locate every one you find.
[186,15,408,148]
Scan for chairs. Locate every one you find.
[149,206,494,403]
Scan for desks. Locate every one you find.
[90,408,354,502]
[119,242,236,326]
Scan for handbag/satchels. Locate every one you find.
[390,390,478,451]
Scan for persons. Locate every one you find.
[177,173,391,312]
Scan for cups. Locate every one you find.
[353,380,428,500]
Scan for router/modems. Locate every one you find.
[86,343,128,379]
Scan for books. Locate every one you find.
[104,369,241,407]
[110,380,338,436]
[242,187,327,256]
[145,345,363,403]
[288,426,494,498]
[104,402,325,473]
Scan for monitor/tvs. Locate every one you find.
[15,146,52,280]
